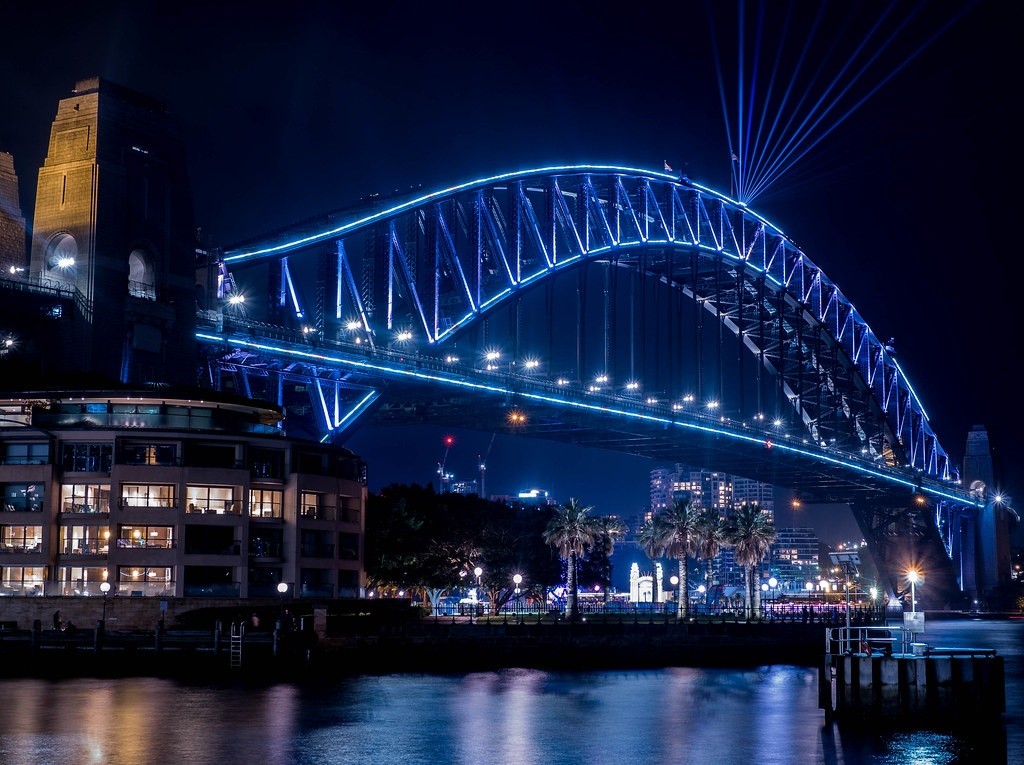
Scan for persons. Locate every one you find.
[53,610,77,631]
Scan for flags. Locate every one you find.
[665,163,673,172]
[731,153,739,162]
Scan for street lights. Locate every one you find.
[762,584,769,619]
[473,567,483,617]
[513,573,523,620]
[806,582,813,607]
[819,580,828,606]
[908,569,917,612]
[670,576,679,617]
[769,577,778,612]
[698,585,706,605]
